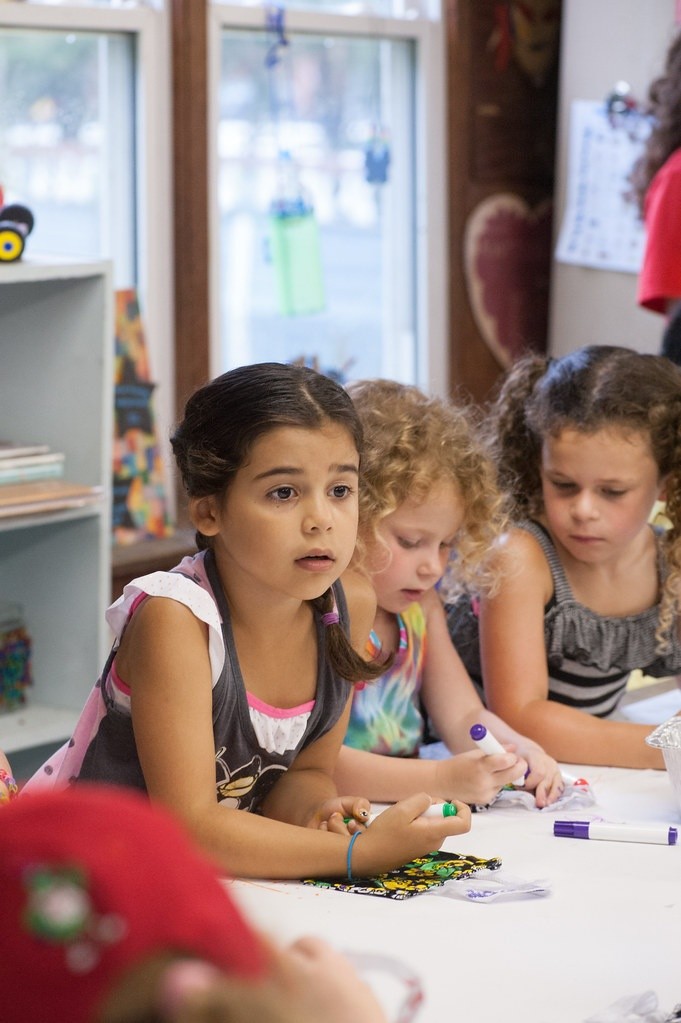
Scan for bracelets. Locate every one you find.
[345,831,362,881]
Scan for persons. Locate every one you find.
[0,39,679,1023]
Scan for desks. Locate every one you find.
[220,686,681,1023]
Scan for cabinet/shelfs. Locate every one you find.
[0,262,111,789]
[112,536,195,603]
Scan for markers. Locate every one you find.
[553,820,678,847]
[470,724,528,789]
[338,801,459,828]
